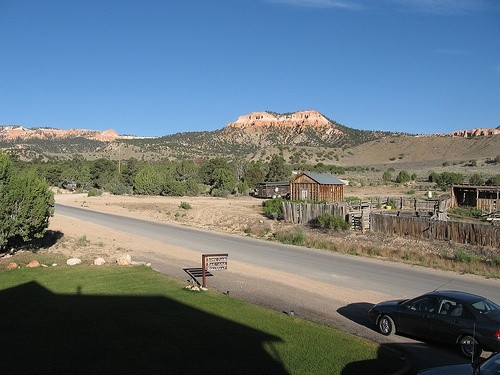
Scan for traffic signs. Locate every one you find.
[205,256,228,270]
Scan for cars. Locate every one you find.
[410,353,500,375]
[368,290,500,359]
